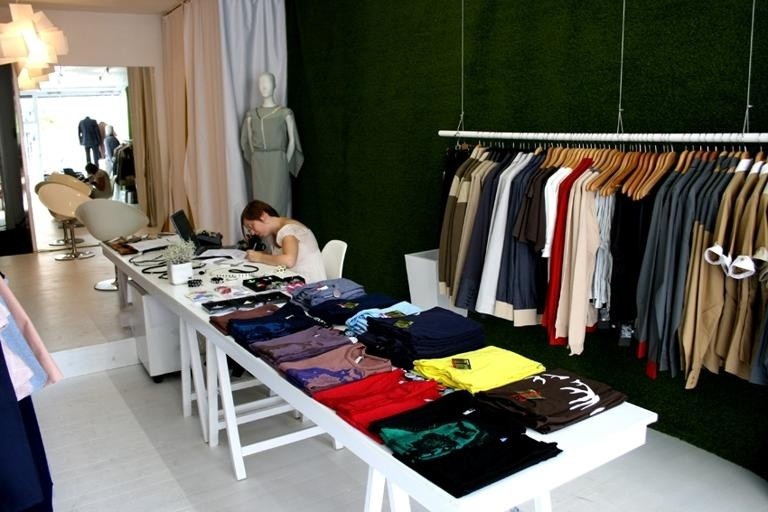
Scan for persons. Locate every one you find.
[96,122,118,160]
[85,162,112,198]
[77,114,102,169]
[241,199,327,284]
[239,72,305,251]
[102,124,120,200]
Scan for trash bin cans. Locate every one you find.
[406,248,469,319]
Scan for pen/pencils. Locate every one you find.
[252,242,258,250]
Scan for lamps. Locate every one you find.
[0,0,68,93]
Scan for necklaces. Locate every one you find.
[129,250,259,281]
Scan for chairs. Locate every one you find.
[33,170,92,260]
[74,199,149,296]
[318,234,353,282]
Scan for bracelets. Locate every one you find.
[186,277,203,287]
[211,277,224,285]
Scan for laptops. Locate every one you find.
[171,209,239,259]
[64,169,78,177]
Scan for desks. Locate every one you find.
[95,229,322,448]
[178,309,660,509]
